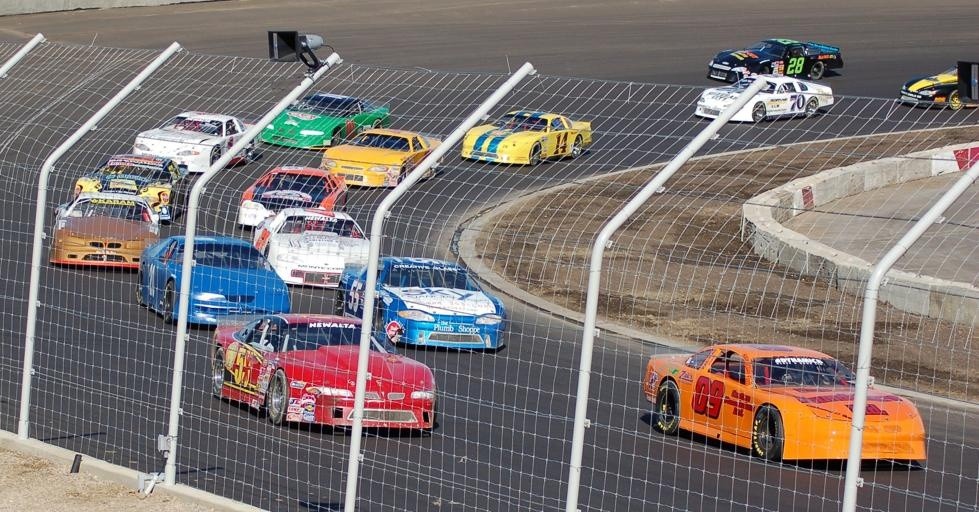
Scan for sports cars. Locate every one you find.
[643,343,928,463]
[899,65,978,110]
[694,38,844,124]
